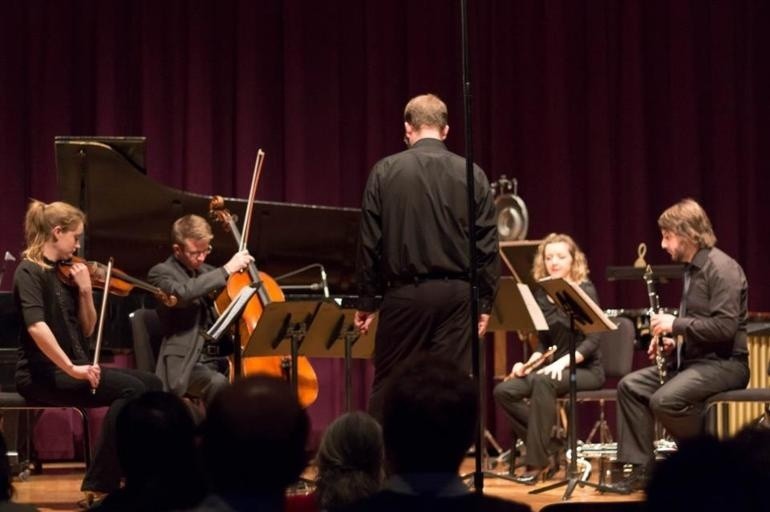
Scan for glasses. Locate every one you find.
[183,246,213,256]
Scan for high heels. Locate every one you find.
[547,444,565,480]
[517,464,550,485]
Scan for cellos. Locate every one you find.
[203,189,323,406]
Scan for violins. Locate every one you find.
[62,254,179,321]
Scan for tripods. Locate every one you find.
[459,331,538,488]
[528,314,630,503]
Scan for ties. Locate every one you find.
[677,270,690,368]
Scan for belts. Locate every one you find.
[201,344,232,357]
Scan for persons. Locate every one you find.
[643,425,770,512]
[489,230,608,495]
[347,92,503,470]
[145,208,257,414]
[83,386,209,511]
[194,368,317,511]
[311,405,388,511]
[349,347,530,512]
[0,423,39,512]
[7,196,168,507]
[599,198,753,498]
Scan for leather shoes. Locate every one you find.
[613,470,650,494]
